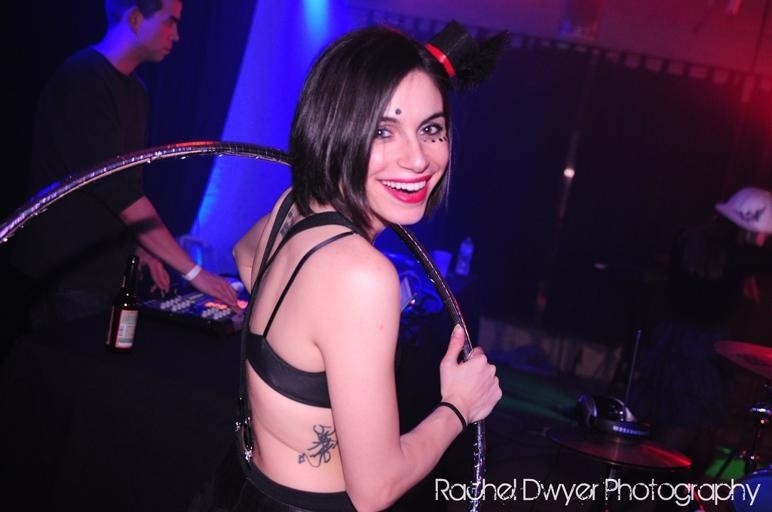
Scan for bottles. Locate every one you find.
[106,255,140,351]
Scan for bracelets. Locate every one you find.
[182,264,204,281]
[437,400,468,434]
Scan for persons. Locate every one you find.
[25,0,244,316]
[229,22,505,510]
